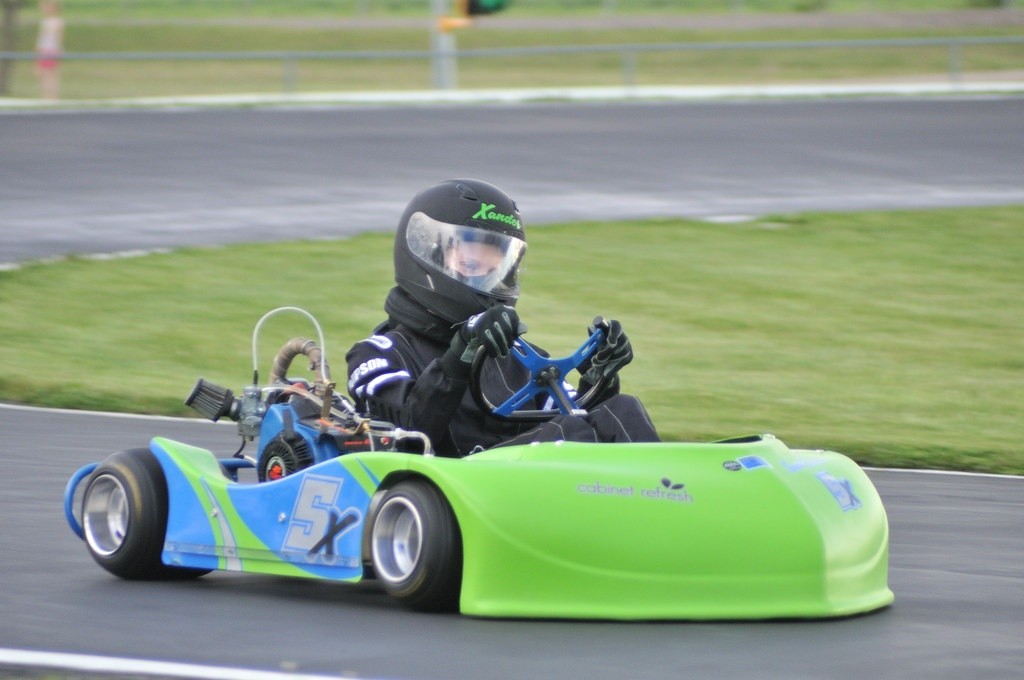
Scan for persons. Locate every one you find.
[36,0,64,99]
[345,178,661,459]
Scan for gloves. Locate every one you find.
[588,320,633,378]
[449,305,528,358]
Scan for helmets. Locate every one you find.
[394,178,527,323]
